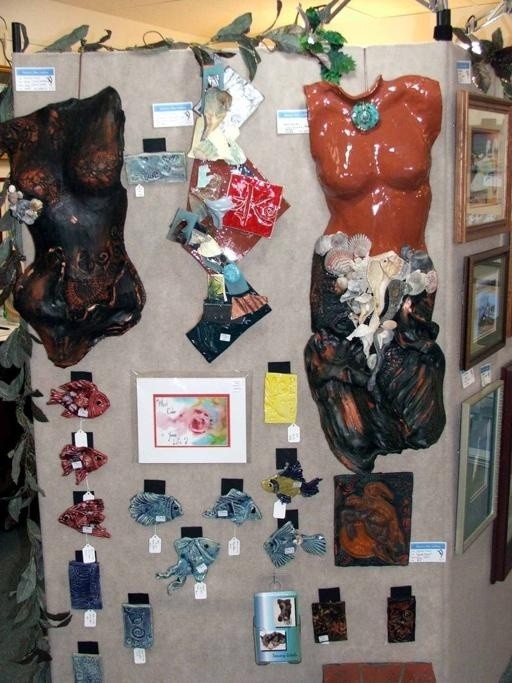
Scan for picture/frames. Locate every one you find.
[455,90,512,584]
